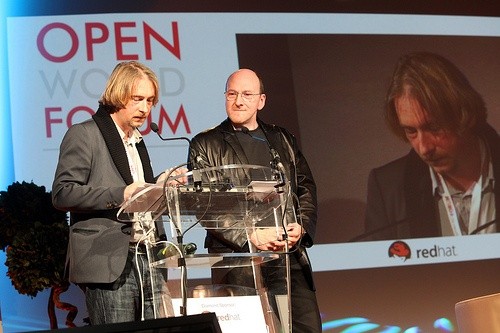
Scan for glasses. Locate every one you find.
[225,89,260,102]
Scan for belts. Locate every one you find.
[129,241,147,251]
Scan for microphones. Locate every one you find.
[241,127,285,188]
[150,122,197,160]
[351,196,441,242]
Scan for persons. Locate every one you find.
[363,50,500,241]
[187,69,322,333]
[51,61,187,324]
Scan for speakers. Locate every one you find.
[27,312,222,333]
